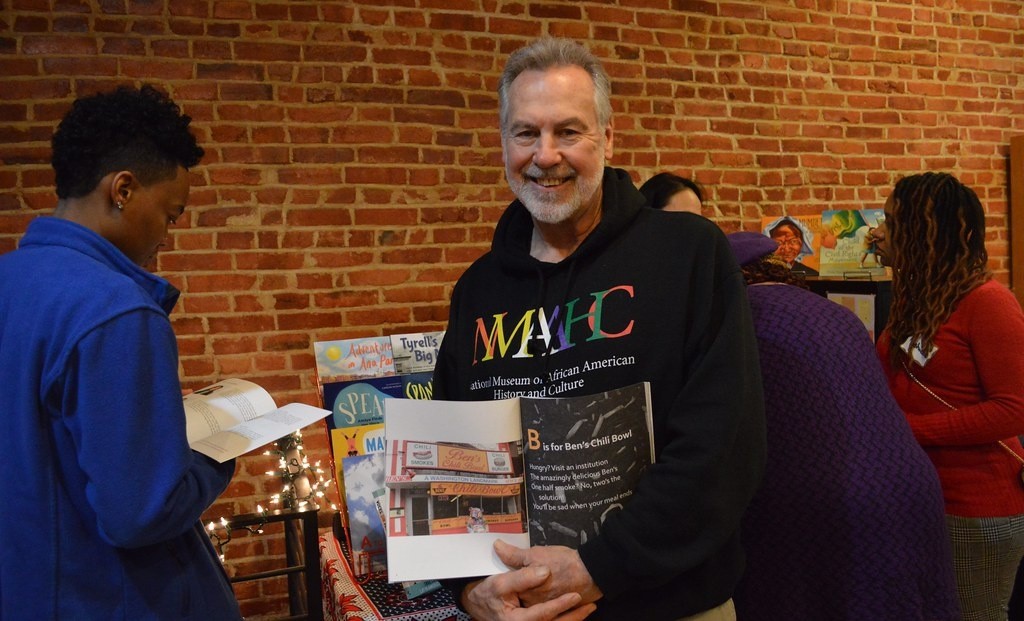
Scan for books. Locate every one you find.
[181,376,335,463]
[315,329,657,599]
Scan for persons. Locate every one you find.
[0,79,248,621]
[639,174,704,216]
[430,37,769,621]
[728,231,966,621]
[195,385,223,396]
[872,172,1024,621]
